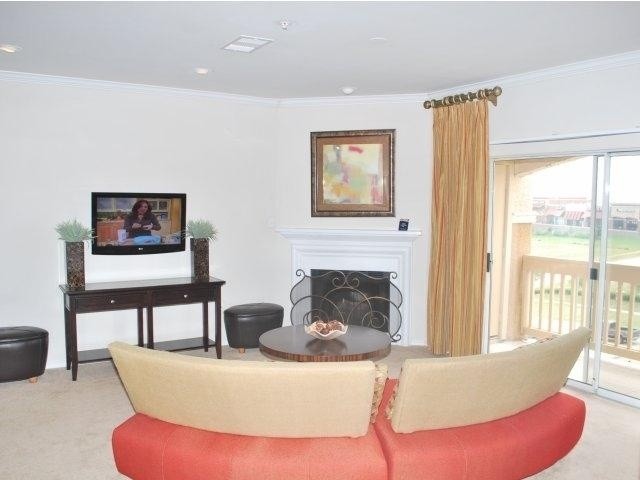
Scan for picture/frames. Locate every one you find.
[310,128,395,217]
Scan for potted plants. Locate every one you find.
[181,219,217,277]
[56,220,96,291]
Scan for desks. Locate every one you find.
[59,279,226,380]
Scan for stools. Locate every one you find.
[223,304,285,354]
[0,327,50,384]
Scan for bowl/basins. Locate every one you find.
[304,320,349,341]
[307,338,347,356]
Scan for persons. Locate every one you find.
[123,200,161,238]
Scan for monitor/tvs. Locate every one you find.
[89,191,187,256]
